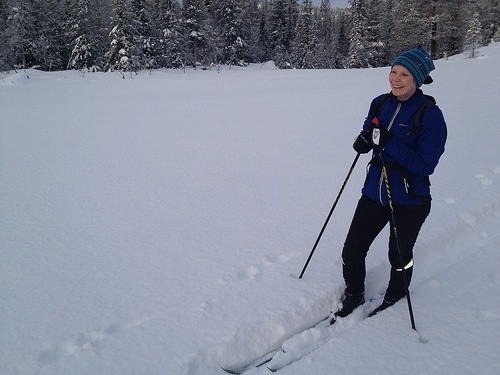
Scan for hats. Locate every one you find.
[392,47,435,87]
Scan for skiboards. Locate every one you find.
[222,282,406,375]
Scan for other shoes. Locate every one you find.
[368,296,399,318]
[334,293,365,317]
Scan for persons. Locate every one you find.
[337,48,447,318]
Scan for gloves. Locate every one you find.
[369,122,393,148]
[353,131,374,154]
